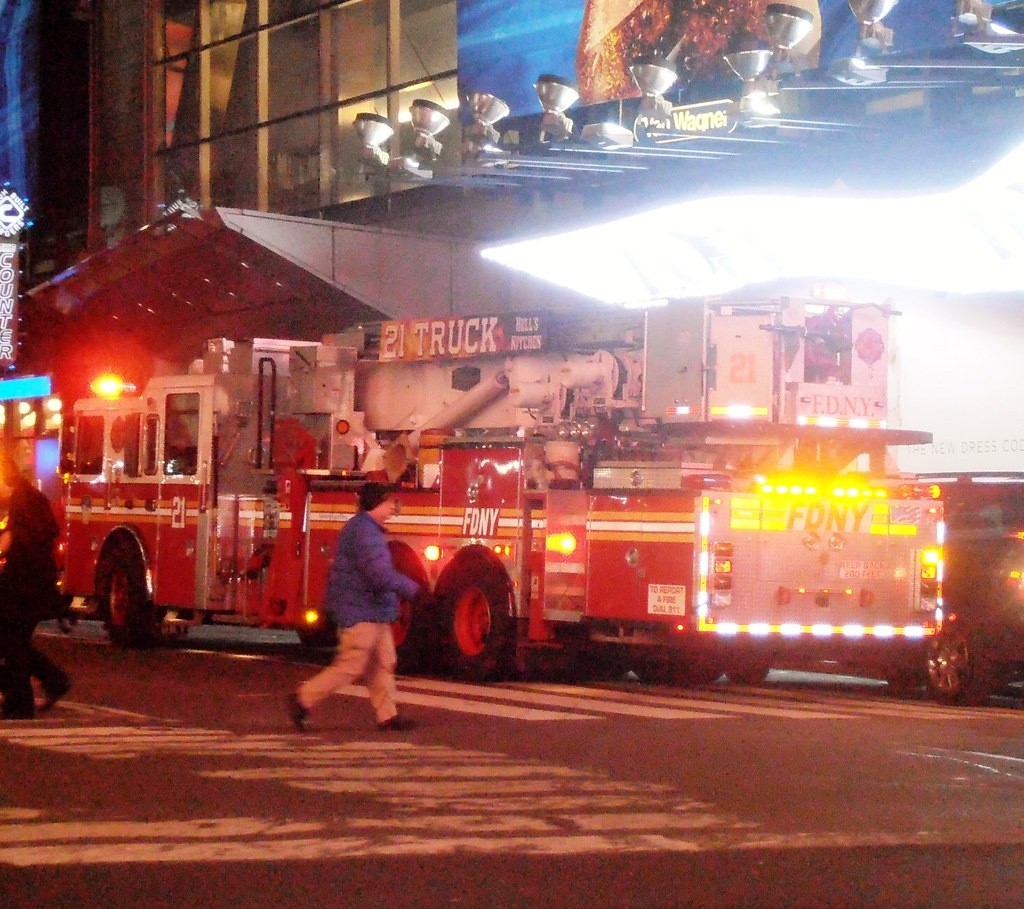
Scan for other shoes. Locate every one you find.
[37,669,70,712]
[378,716,415,731]
[288,693,309,732]
[0,701,34,719]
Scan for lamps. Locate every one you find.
[949,0,994,26]
[848,0,900,48]
[534,74,581,137]
[466,91,510,144]
[409,97,451,155]
[724,50,781,100]
[629,63,679,119]
[767,2,815,50]
[353,112,396,167]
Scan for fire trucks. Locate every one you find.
[64,281,1024,704]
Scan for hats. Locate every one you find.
[358,483,389,511]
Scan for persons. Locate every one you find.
[0,457,73,719]
[286,482,421,732]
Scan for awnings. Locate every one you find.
[26,200,625,365]
